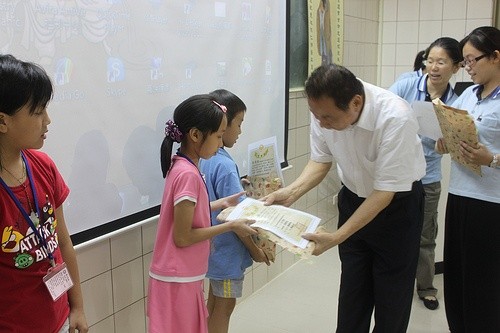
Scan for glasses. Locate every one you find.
[459,53,488,66]
[422,60,445,68]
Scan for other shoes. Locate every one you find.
[423,297,439,310]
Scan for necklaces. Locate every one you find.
[2,159,40,228]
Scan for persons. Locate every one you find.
[388,37,464,310]
[0,54,89,333]
[146,95,258,333]
[257,63,426,333]
[198,89,271,333]
[434,26,500,333]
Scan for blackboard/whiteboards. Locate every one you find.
[287,0,309,94]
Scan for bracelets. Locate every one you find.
[488,155,497,169]
